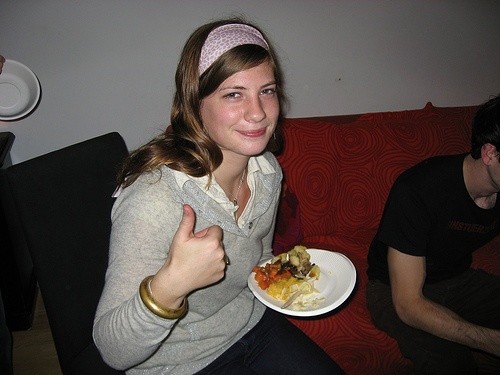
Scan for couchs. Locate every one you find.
[271,104,500,374]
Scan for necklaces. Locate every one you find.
[212,166,250,205]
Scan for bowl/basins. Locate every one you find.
[1,60,41,121]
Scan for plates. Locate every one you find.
[249,247,356,318]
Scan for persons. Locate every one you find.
[363,93,500,375]
[90,17,349,375]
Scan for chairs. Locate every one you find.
[0,131,132,374]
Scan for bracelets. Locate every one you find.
[138,275,188,320]
